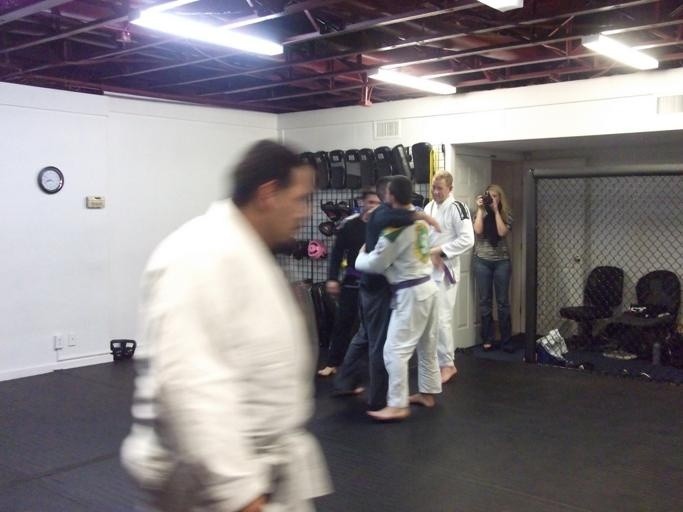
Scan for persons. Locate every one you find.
[471,184,519,354]
[114,135,337,512]
[353,175,442,422]
[331,177,442,396]
[314,190,383,376]
[422,169,476,387]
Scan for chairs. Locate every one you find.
[559,266,681,359]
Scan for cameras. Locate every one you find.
[481,191,493,206]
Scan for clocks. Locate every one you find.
[37,167,63,193]
